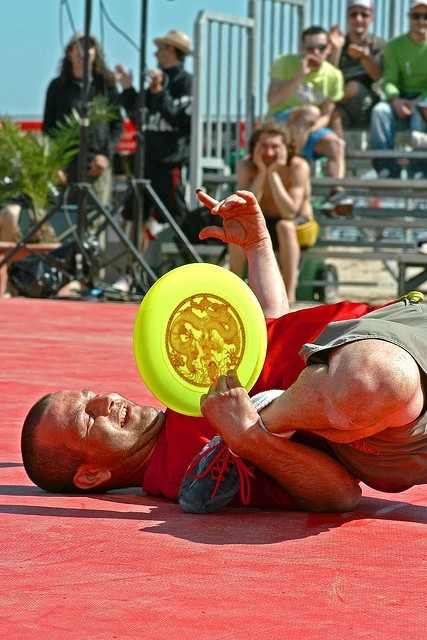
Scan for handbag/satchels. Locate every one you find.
[179,206,226,244]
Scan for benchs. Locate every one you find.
[202,121,427,298]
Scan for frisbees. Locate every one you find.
[133,261,269,417]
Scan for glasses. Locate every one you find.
[410,12,427,20]
[303,43,328,51]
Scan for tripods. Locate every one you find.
[53,0,205,274]
[1,0,158,284]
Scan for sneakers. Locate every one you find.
[177,388,298,515]
[112,271,139,296]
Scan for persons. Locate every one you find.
[229,118,320,308]
[267,25,353,217]
[112,29,194,297]
[41,32,123,279]
[20,190,427,511]
[369,0,427,179]
[328,0,387,140]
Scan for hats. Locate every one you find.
[410,1,427,10]
[154,29,191,54]
[346,1,374,11]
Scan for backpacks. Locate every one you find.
[7,244,77,299]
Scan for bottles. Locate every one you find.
[375,200,405,209]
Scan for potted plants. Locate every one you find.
[0,96,123,299]
